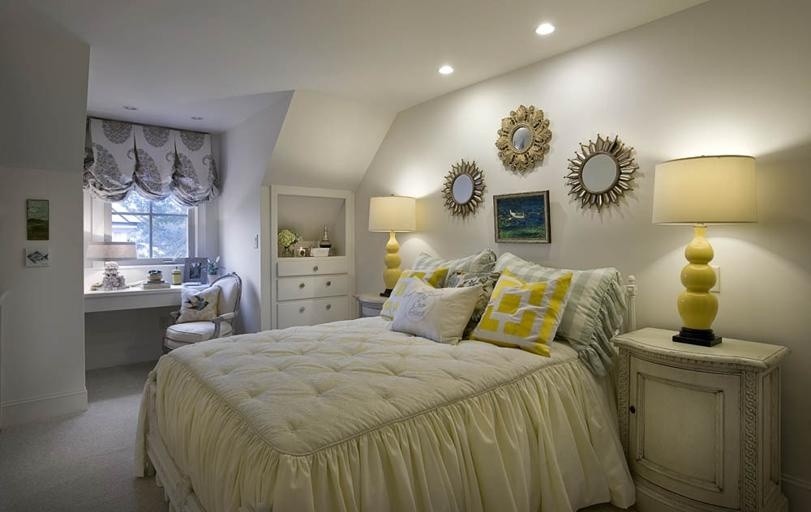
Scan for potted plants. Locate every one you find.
[205,257,221,283]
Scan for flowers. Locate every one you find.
[278,228,298,248]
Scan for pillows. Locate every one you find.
[382,251,627,377]
[176,284,222,323]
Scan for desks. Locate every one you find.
[84,280,181,317]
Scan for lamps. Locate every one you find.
[366,191,418,296]
[649,149,759,348]
[86,239,140,291]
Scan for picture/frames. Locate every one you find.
[494,189,552,245]
[181,257,207,284]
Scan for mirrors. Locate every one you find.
[441,157,489,220]
[494,104,554,173]
[563,132,640,211]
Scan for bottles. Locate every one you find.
[319,223,333,248]
[171,269,182,286]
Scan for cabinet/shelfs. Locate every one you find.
[275,256,351,328]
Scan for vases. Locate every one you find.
[283,246,293,257]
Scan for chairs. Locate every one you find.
[160,271,243,353]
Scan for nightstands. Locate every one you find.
[353,290,385,318]
[612,326,791,512]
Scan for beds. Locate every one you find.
[134,253,642,512]
